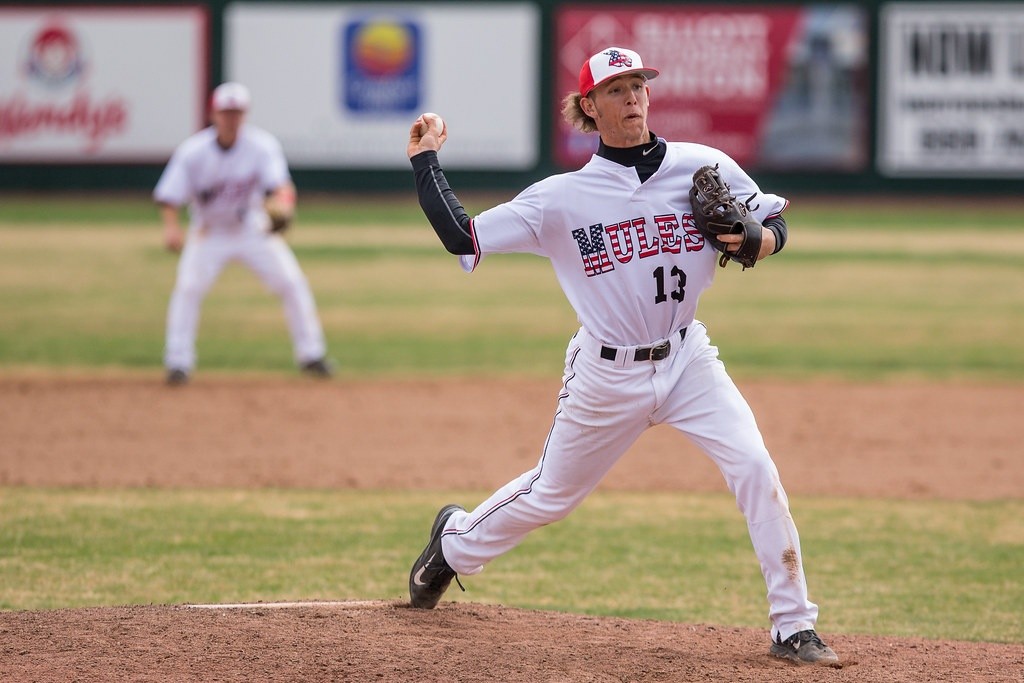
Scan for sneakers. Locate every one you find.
[771,628,838,665]
[409,505,466,608]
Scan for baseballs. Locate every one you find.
[417,112,443,138]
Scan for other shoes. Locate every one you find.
[167,367,188,385]
[302,361,331,380]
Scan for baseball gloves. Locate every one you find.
[263,192,294,234]
[689,165,763,270]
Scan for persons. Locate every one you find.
[154,81,334,387]
[406,46,842,670]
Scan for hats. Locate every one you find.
[578,47,660,98]
[213,83,246,110]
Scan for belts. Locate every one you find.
[601,327,688,361]
